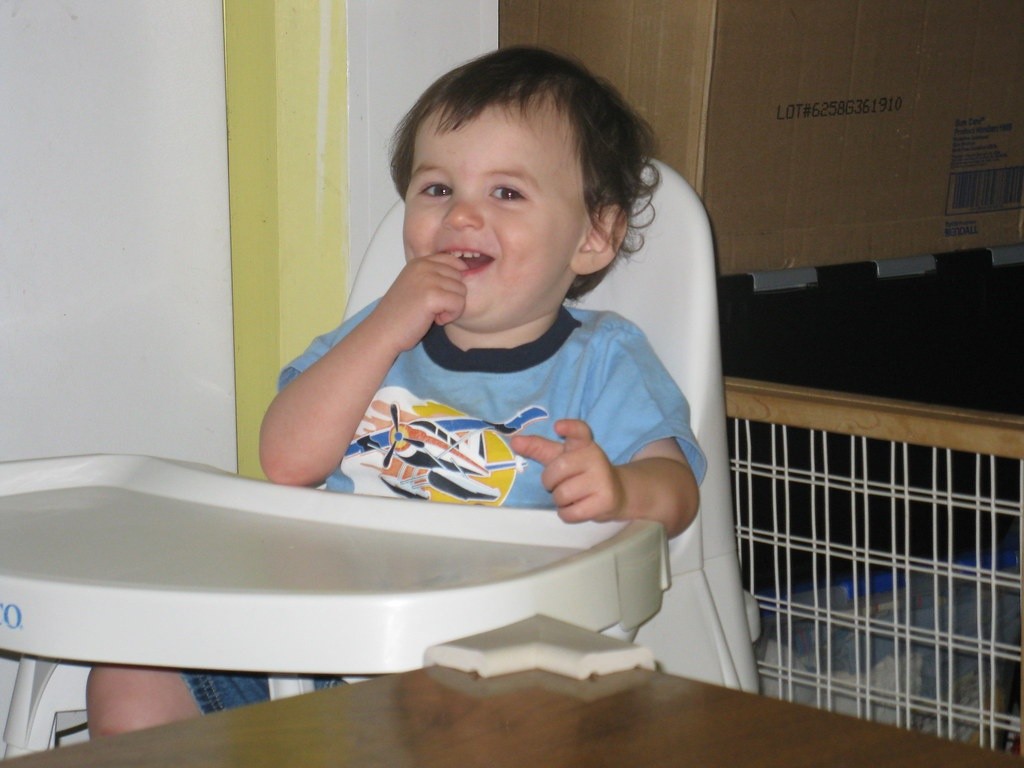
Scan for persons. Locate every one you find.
[86,46,706,740]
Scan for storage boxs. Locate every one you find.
[498,0,1024,276]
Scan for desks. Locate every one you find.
[0,613,1024,768]
[0,453,674,677]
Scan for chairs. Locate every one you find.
[267,157,762,704]
